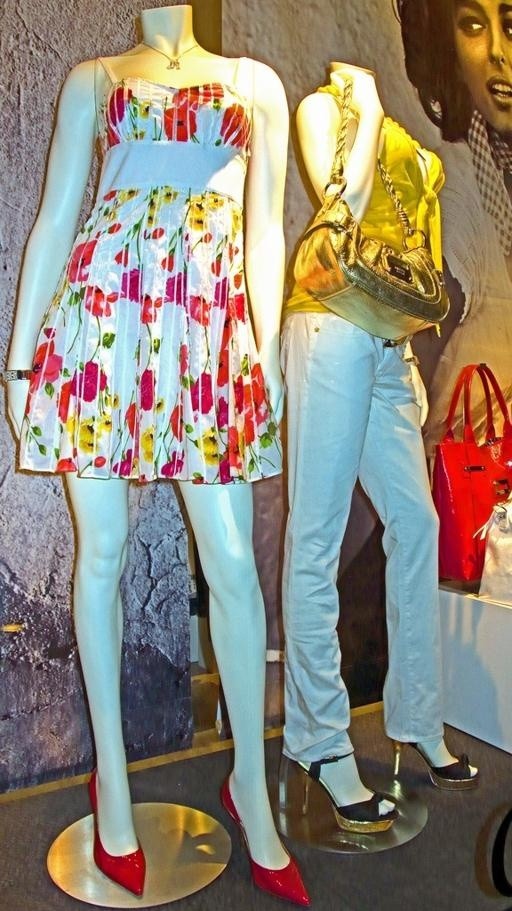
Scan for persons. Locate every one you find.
[6,3,314,908]
[279,58,482,833]
[393,1,512,162]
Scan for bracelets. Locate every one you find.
[2,369,32,384]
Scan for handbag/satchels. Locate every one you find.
[474,502,512,606]
[430,360,512,584]
[328,98,449,323]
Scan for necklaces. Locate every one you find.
[137,38,203,65]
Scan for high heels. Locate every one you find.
[362,794,370,796]
[220,778,311,906]
[283,744,399,834]
[86,769,147,897]
[389,735,480,790]
